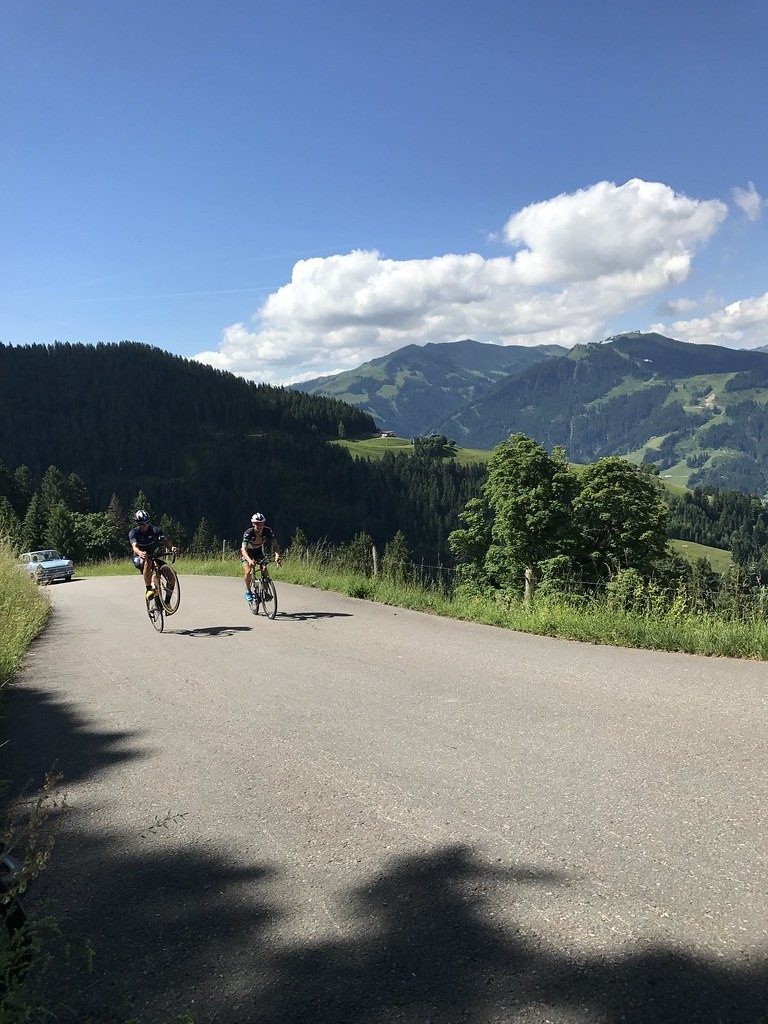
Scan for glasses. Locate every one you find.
[138,520,150,526]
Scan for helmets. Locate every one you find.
[251,513,265,522]
[134,510,150,520]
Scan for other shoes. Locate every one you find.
[266,594,273,602]
[245,591,253,601]
[146,590,154,600]
[164,601,174,613]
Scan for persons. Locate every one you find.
[241,513,282,601]
[33,556,37,562]
[44,554,49,559]
[129,509,179,613]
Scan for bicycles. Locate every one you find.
[244,559,283,621]
[138,550,183,632]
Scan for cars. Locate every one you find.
[16,549,75,586]
